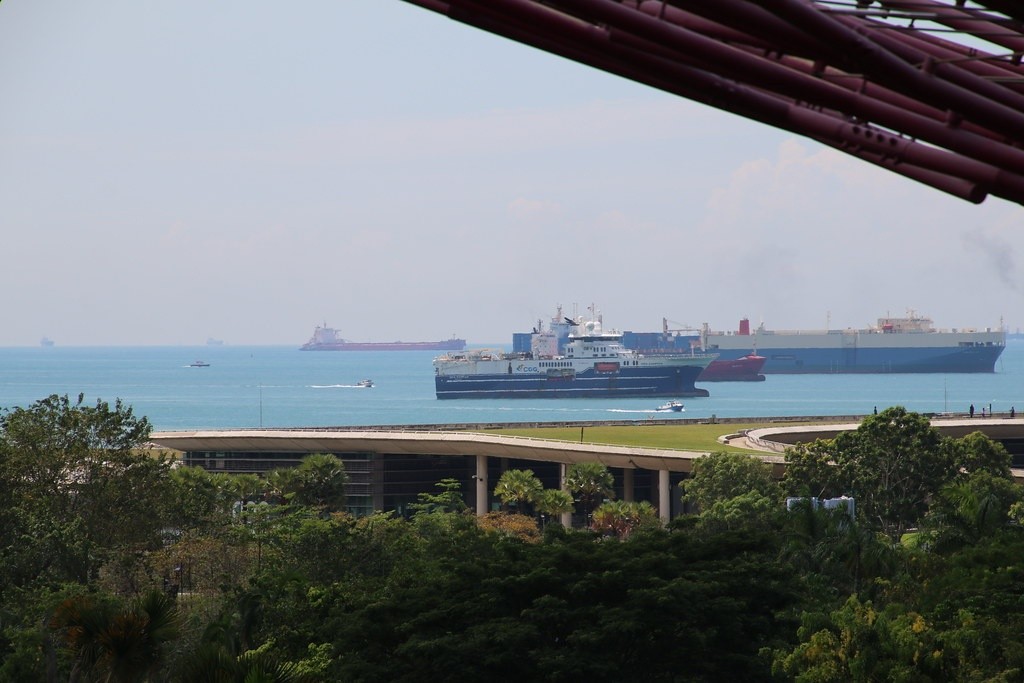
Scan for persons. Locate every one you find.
[982,408,987,417]
[874,406,878,414]
[970,404,974,417]
[1010,407,1015,417]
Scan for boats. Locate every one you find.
[511,316,1007,373]
[297,321,466,352]
[190,360,209,367]
[654,401,685,412]
[695,353,767,381]
[356,379,374,387]
[433,302,721,400]
